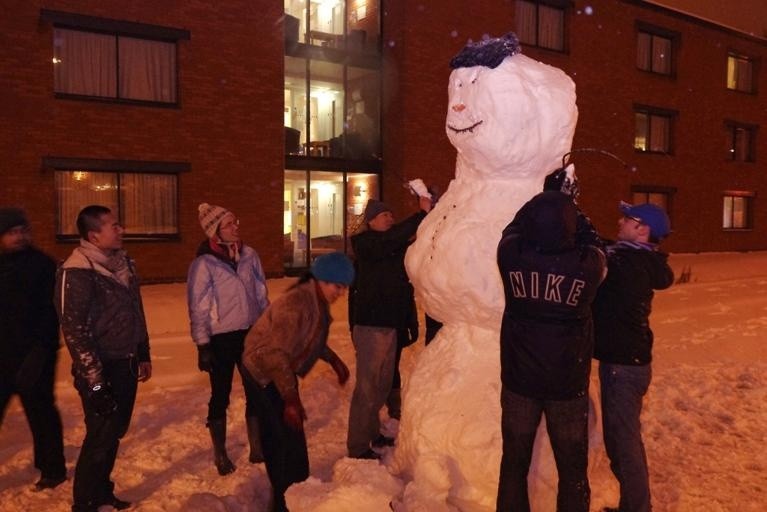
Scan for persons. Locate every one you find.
[495,168,609,511]
[562,176,675,512]
[51,204,151,510]
[187,202,270,476]
[0,207,67,490]
[343,178,443,458]
[238,250,355,512]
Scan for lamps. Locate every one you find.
[353,185,367,197]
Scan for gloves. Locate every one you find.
[198,347,213,372]
[88,379,117,417]
[285,394,306,431]
[331,357,350,384]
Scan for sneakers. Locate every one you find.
[37,475,66,489]
[374,436,394,447]
[360,450,380,460]
[73,497,129,512]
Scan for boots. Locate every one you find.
[245,414,264,463]
[203,419,233,475]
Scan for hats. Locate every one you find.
[365,198,390,222]
[1,207,27,235]
[619,200,667,237]
[200,202,235,238]
[311,253,353,284]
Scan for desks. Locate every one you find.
[304,30,344,45]
[303,141,329,157]
[303,249,336,263]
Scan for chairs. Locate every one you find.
[285,12,300,41]
[284,126,300,154]
